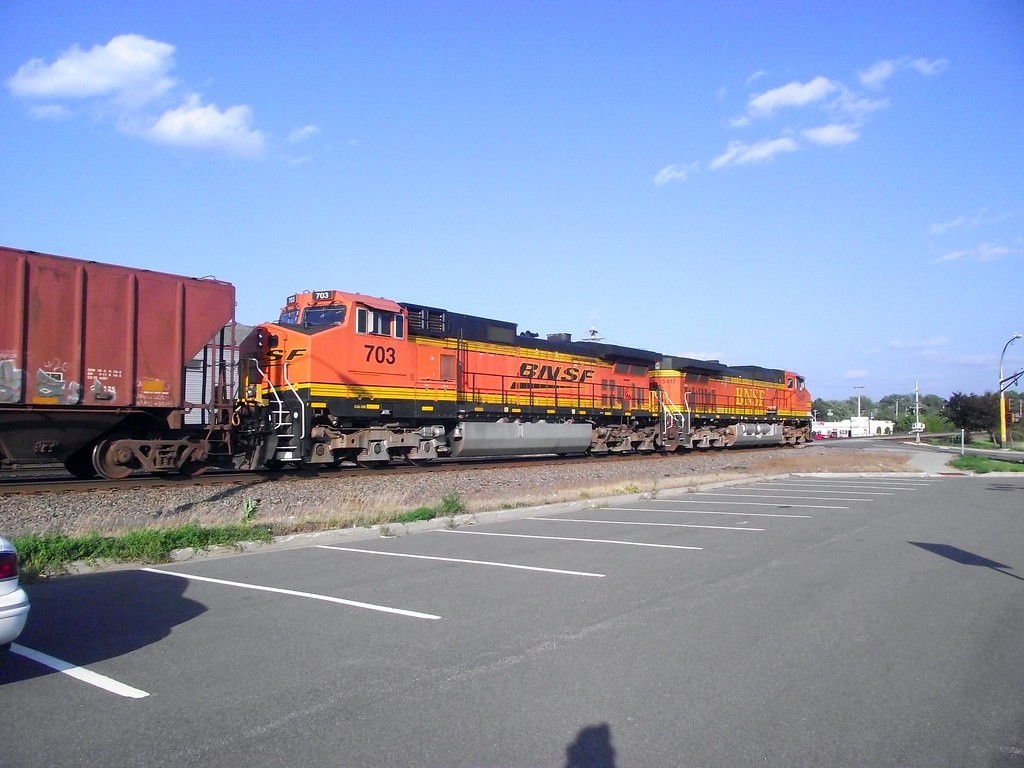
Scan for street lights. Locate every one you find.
[999,333,1022,448]
[852,385,864,417]
[0,245,815,483]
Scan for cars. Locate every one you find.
[0,536,31,657]
